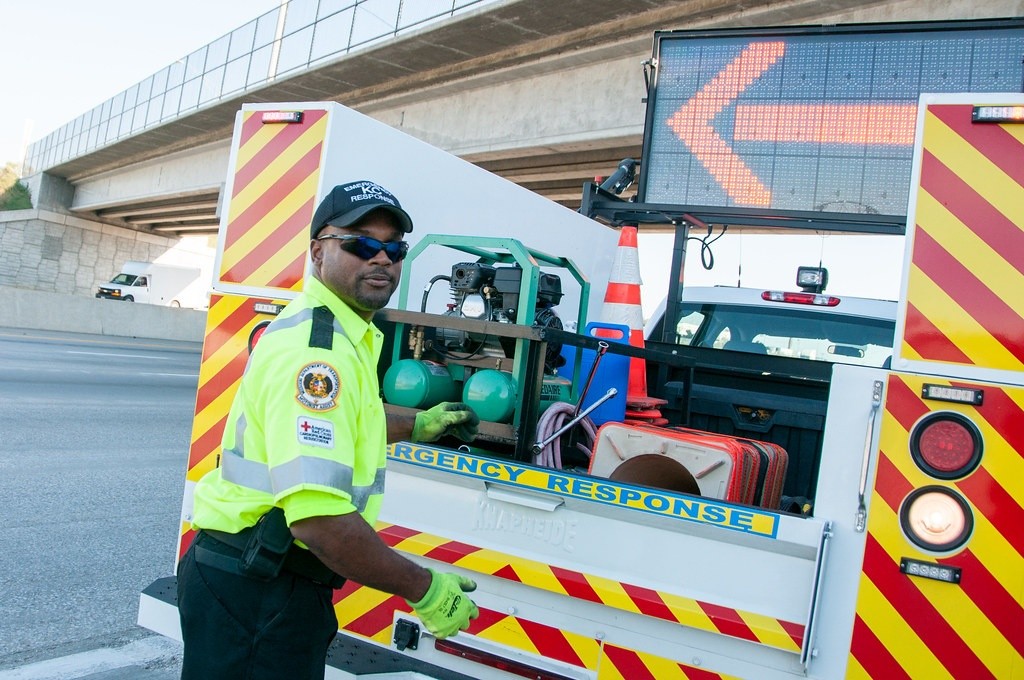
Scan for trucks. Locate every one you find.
[94,261,205,310]
[138,6,1024,680]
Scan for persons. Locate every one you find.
[177,182,479,680]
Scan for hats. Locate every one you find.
[310,180,413,241]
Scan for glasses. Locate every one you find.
[317,234,409,264]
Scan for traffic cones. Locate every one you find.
[592,220,669,427]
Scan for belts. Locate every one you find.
[203,529,347,590]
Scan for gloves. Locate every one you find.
[411,401,479,443]
[405,567,479,639]
